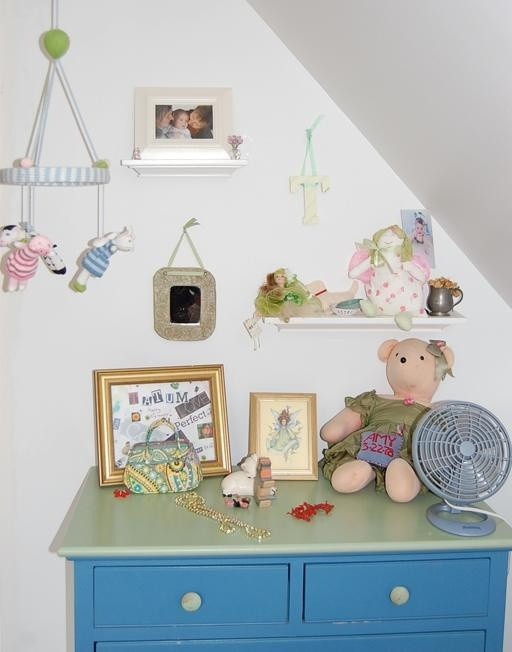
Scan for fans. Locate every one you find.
[412,400,512,537]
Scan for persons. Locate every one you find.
[155,105,176,138]
[244,269,322,350]
[185,106,213,139]
[168,110,190,138]
[407,219,431,259]
[2,225,67,274]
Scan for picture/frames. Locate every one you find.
[249,392,318,481]
[93,364,232,487]
[134,86,234,148]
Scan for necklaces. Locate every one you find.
[178,488,273,540]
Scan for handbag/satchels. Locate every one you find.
[123,419,201,495]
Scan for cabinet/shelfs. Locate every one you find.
[58,466,512,651]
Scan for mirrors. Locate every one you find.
[170,286,200,324]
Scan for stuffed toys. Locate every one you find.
[318,338,455,503]
[348,225,431,331]
[5,236,51,292]
[222,454,278,496]
[75,227,130,293]
[256,457,272,507]
[304,280,360,314]
